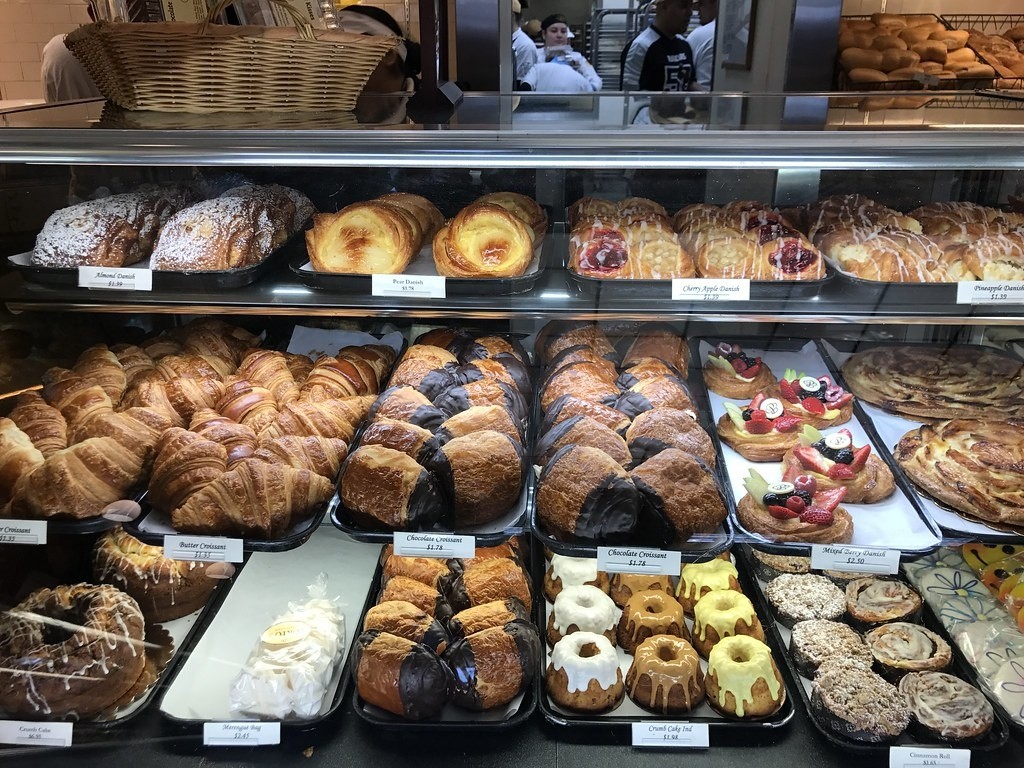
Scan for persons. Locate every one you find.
[509,0,717,94]
[350,92,414,127]
[40,33,105,105]
[338,4,426,94]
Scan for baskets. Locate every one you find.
[64,0,403,114]
[90,100,365,130]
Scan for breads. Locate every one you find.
[0,14,1024,746]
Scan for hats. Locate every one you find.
[512,0,521,13]
[540,13,567,28]
[567,27,575,38]
[337,11,407,63]
[525,19,542,37]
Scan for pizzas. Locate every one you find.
[894,420,1024,525]
[841,346,1024,420]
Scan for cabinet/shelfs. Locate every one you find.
[0,89,1024,768]
